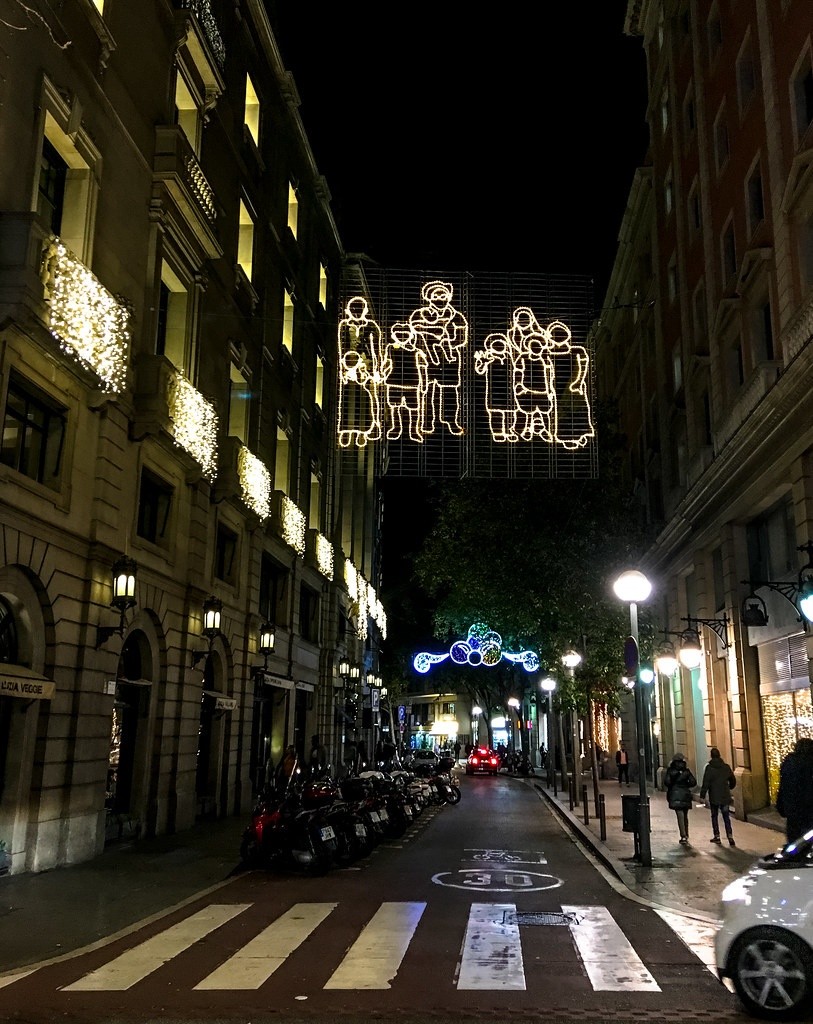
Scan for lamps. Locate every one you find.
[333,655,388,704]
[190,595,224,670]
[640,612,730,683]
[95,555,137,650]
[248,622,276,680]
[741,539,813,627]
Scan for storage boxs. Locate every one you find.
[302,762,433,812]
[439,758,456,770]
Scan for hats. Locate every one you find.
[711,748,720,758]
[672,753,684,760]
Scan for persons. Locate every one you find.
[699,748,736,845]
[340,735,395,771]
[309,735,328,771]
[540,742,547,769]
[616,744,630,787]
[454,742,461,762]
[595,743,602,761]
[465,742,471,755]
[664,753,697,844]
[497,742,505,757]
[775,738,813,845]
[283,745,298,785]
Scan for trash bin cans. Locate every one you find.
[622,795,652,834]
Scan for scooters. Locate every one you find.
[515,749,534,773]
[240,742,461,868]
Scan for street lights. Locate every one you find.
[562,650,582,808]
[541,678,559,797]
[509,697,517,774]
[466,706,482,744]
[615,572,650,868]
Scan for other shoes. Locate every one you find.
[729,837,735,845]
[710,836,721,844]
[627,784,630,787]
[679,838,687,844]
[619,782,622,788]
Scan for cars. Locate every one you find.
[408,749,438,769]
[466,749,497,776]
[711,829,813,1024]
